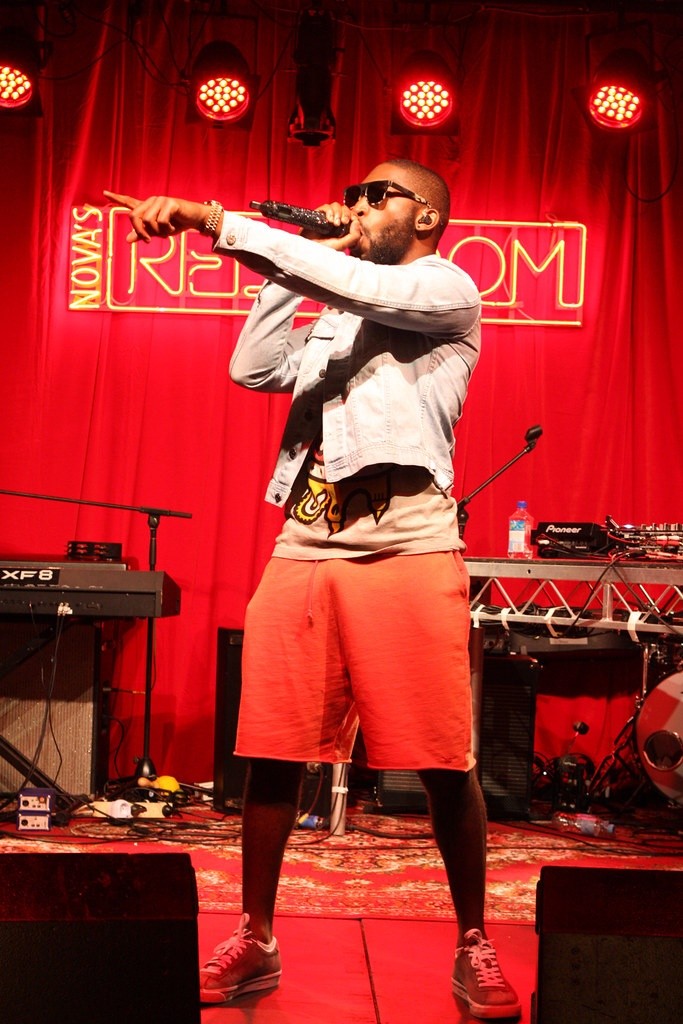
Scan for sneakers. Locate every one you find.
[199,935,283,1003]
[450,942,521,1018]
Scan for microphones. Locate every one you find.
[249,200,353,237]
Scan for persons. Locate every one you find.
[97,157,522,1020]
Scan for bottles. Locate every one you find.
[552,811,614,837]
[294,810,329,828]
[508,501,534,559]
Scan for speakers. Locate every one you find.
[377,648,538,814]
[0,615,117,799]
[531,865,683,1024]
[0,851,200,1024]
[217,627,357,819]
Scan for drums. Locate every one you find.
[634,668,683,802]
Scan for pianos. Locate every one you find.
[0,554,186,622]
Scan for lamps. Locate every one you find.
[0,0,51,119]
[570,0,661,143]
[181,0,260,134]
[391,0,474,136]
[288,1,347,146]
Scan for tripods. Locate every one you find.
[0,489,214,798]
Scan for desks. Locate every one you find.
[460,555,683,831]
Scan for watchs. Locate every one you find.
[196,196,225,233]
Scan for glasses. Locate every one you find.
[343,180,430,207]
